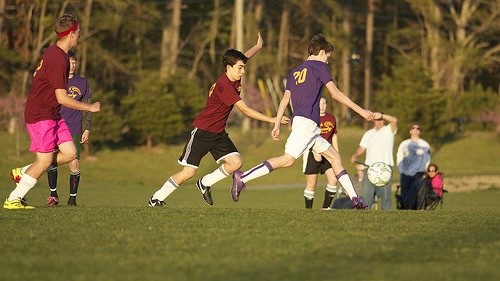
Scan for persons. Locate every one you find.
[147,32,290,208]
[3,13,102,210]
[46,50,93,208]
[396,124,431,209]
[232,33,375,210]
[351,108,397,210]
[418,163,443,210]
[302,94,340,210]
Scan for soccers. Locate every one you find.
[367,162,393,187]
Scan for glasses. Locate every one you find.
[412,127,420,130]
[429,169,436,172]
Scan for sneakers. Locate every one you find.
[11,166,28,199]
[4,198,36,210]
[148,196,166,206]
[67,198,77,206]
[230,170,246,202]
[195,177,214,206]
[46,196,59,207]
[351,196,368,210]
